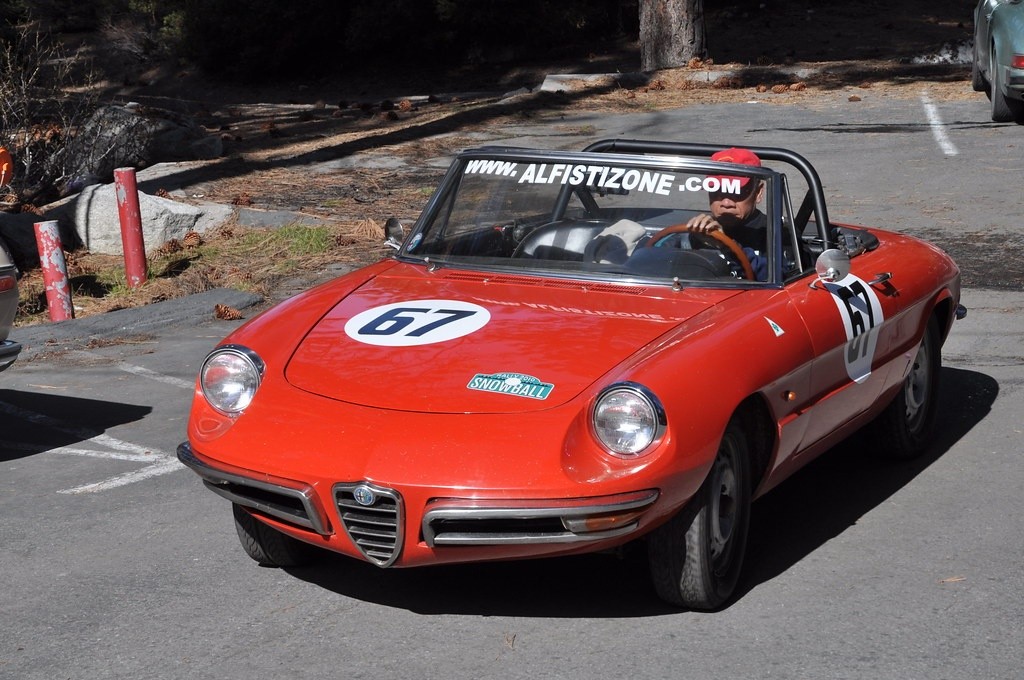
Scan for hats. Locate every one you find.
[700,147,762,190]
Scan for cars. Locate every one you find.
[971,0,1024,125]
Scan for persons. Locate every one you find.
[630,147,815,282]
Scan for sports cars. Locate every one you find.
[173,136,968,614]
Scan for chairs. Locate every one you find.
[510,220,612,263]
